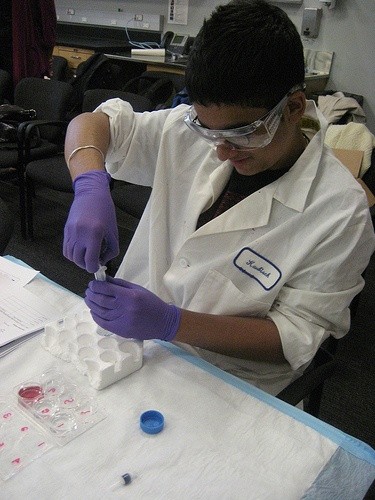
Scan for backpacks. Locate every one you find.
[121,75,176,110]
[68,51,114,119]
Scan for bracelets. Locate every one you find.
[68,146,104,173]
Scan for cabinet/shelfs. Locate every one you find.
[50,39,159,79]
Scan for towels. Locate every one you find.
[316,90,375,179]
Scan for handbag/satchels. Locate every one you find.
[0,103,42,149]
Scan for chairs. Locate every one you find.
[0,56,188,276]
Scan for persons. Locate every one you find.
[65,1,375,413]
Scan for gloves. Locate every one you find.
[63,170,120,274]
[84,275,181,342]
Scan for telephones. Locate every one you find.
[160,30,191,56]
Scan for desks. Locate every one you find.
[0,254,375,500]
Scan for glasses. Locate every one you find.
[183,83,307,153]
